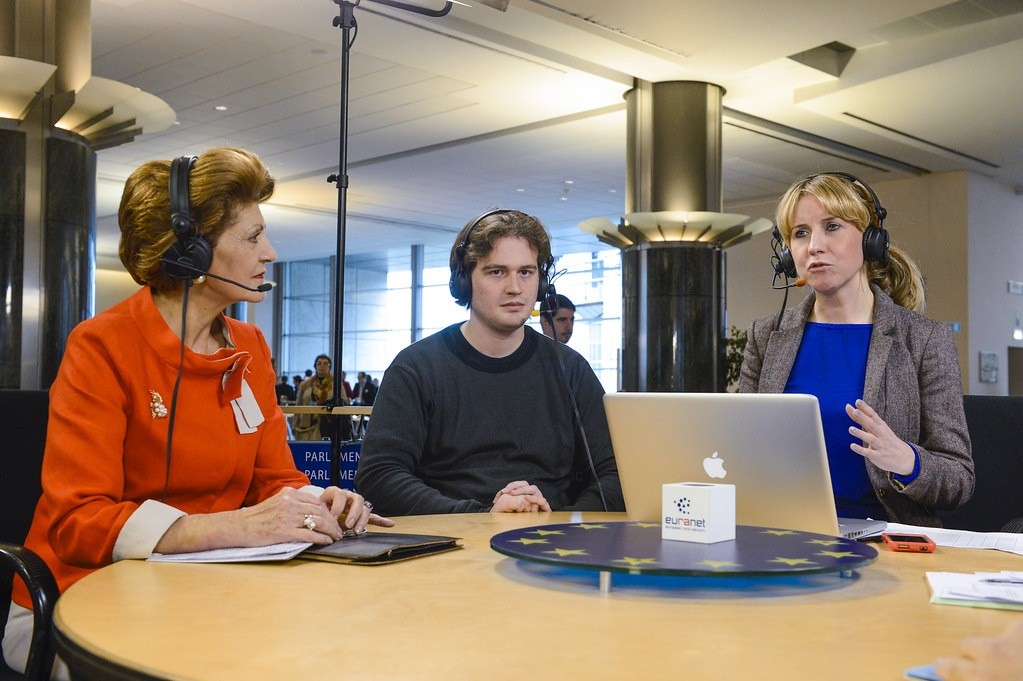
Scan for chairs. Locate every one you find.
[941,394,1023,534]
[0,389,60,681]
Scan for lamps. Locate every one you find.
[579,212,774,251]
[0,55,176,151]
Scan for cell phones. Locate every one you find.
[883,532,935,551]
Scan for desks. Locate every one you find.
[50,511,1023,680]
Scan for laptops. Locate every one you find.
[604,393,887,540]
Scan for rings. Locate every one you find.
[364,500,373,511]
[304,514,316,530]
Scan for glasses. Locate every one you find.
[316,361,330,367]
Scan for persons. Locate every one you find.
[540,294,576,343]
[272,354,378,441]
[1,146,395,681]
[354,212,624,515]
[738,173,976,530]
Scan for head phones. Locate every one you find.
[450,209,559,307]
[770,172,890,279]
[161,155,215,280]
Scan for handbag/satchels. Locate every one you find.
[294,423,321,441]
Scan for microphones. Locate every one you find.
[531,297,558,316]
[770,274,806,289]
[190,268,273,292]
